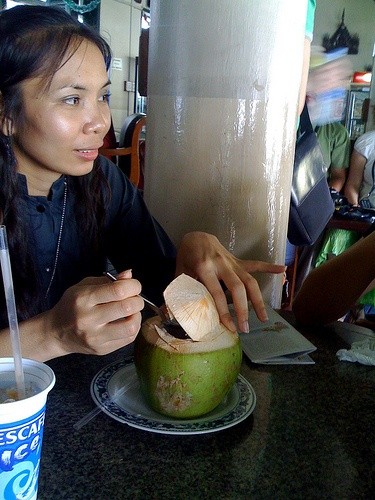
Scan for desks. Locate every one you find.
[38,310,375,500]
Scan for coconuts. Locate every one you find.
[131,273,242,418]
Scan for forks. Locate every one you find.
[104,271,178,326]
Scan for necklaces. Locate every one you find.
[47,177,68,295]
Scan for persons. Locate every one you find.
[0,6,287,364]
[286,122,375,331]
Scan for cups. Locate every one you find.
[0,355,56,500]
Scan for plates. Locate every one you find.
[89,354,258,436]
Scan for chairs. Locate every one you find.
[98,112,147,188]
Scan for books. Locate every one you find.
[227,302,317,365]
